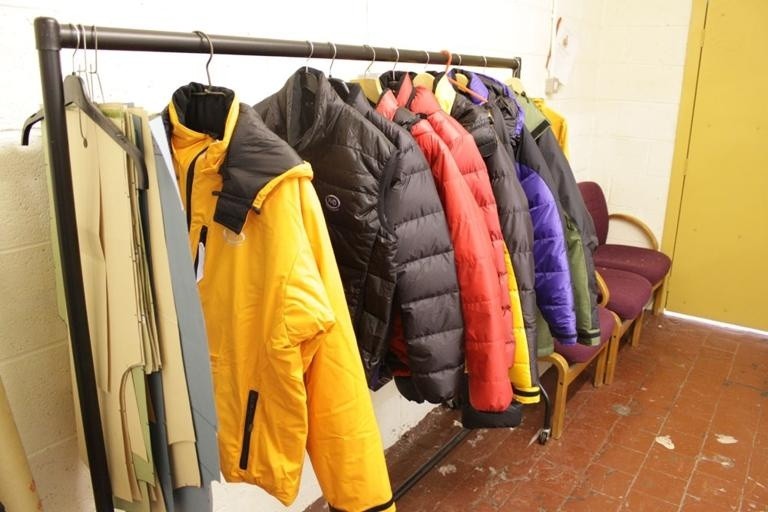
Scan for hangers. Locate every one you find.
[188,27,226,109]
[298,38,521,125]
[19,23,151,193]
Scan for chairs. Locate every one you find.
[579,180,672,347]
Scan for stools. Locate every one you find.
[536,264,655,441]
